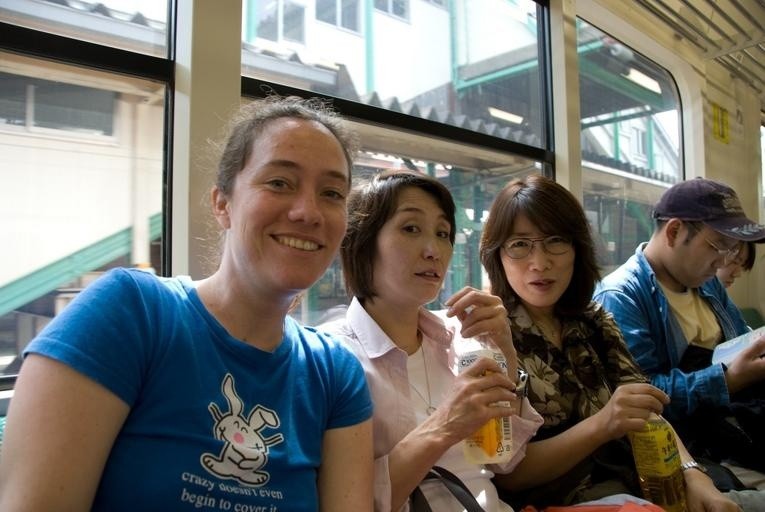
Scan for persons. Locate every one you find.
[0,98,374,512]
[478,175,764,511]
[312,169,664,512]
[715,240,756,288]
[591,174,765,470]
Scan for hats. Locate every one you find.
[651,175,765,246]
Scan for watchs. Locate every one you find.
[680,461,707,473]
[513,367,531,399]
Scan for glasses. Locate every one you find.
[691,225,741,263]
[502,231,571,257]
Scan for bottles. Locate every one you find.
[631,407,688,512]
[451,324,515,489]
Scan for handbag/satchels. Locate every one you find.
[663,343,765,475]
[619,455,757,496]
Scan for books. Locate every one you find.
[712,325,765,366]
